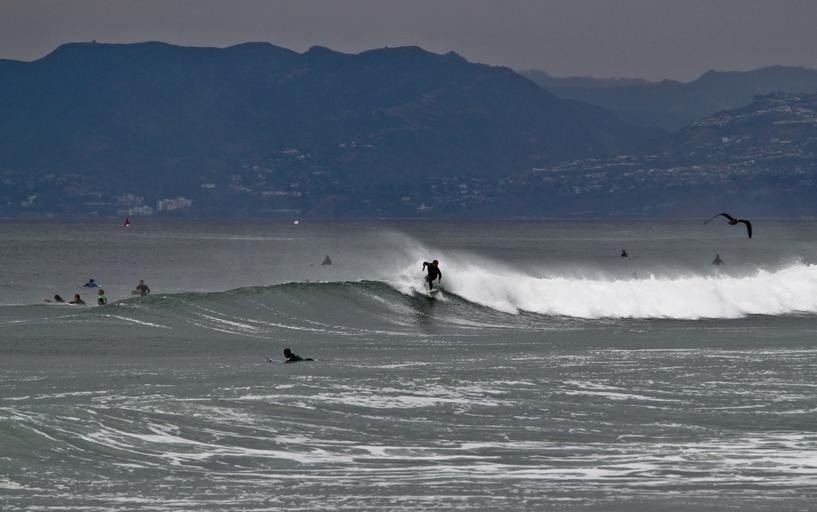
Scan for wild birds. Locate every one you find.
[703,212,752,239]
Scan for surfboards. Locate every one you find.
[427,289,438,298]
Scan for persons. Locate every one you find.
[136,279,150,296]
[54,294,64,302]
[283,348,314,363]
[96,288,107,305]
[83,278,98,288]
[620,249,628,257]
[711,253,725,266]
[422,259,441,290]
[322,255,331,265]
[68,294,86,305]
